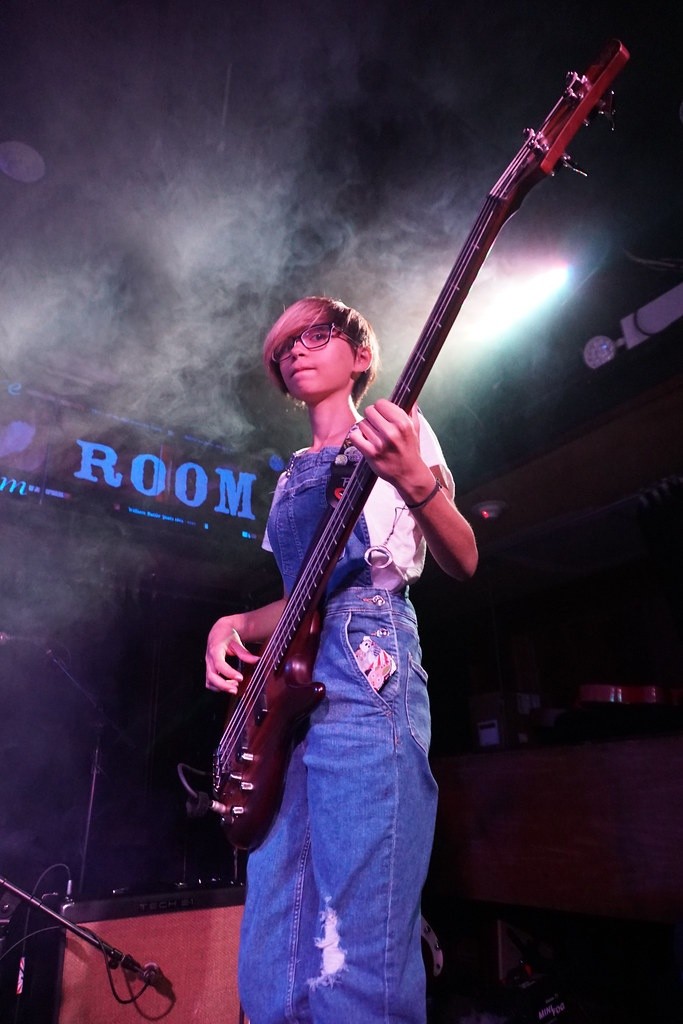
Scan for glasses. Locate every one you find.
[270,321,359,363]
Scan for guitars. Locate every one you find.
[173,34,631,851]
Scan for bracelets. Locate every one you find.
[405,478,443,510]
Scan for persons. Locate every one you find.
[204,295,479,1024]
